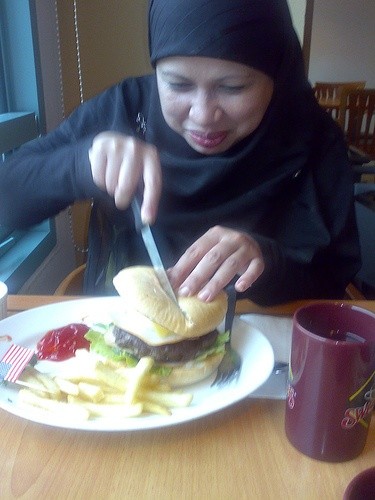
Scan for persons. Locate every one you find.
[0,0,362,307]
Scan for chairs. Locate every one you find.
[312,82,375,161]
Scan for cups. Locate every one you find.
[343,467,375,500]
[285,302,375,463]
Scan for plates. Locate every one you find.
[0,296,274,432]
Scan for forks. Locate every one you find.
[211,286,242,386]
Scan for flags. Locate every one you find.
[0,342,36,383]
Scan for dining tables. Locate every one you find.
[1,295,375,500]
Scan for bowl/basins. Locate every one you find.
[0,281,8,321]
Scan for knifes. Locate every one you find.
[130,195,177,304]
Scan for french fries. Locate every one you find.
[14,350,193,416]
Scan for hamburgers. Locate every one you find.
[81,265,231,386]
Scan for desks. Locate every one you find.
[317,98,373,109]
